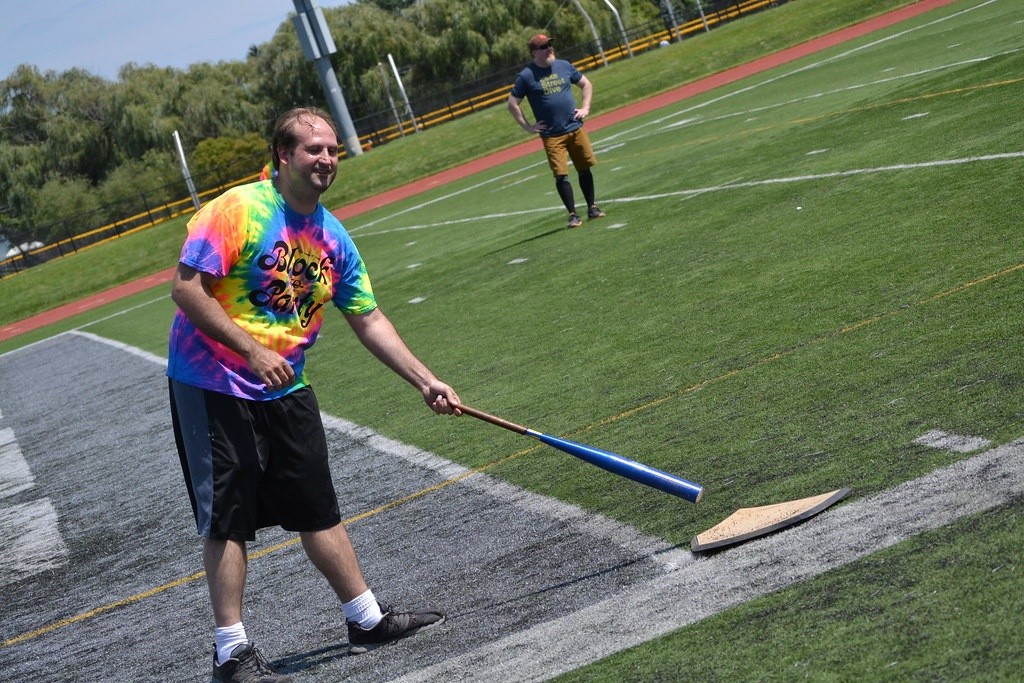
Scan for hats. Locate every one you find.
[527,33,555,50]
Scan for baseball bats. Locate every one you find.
[449,403,704,504]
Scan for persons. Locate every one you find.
[165,108,463,683]
[507,34,605,227]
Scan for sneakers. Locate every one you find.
[344,601,447,654]
[210,641,293,683]
[587,206,606,218]
[567,212,582,227]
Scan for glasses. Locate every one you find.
[537,42,552,50]
